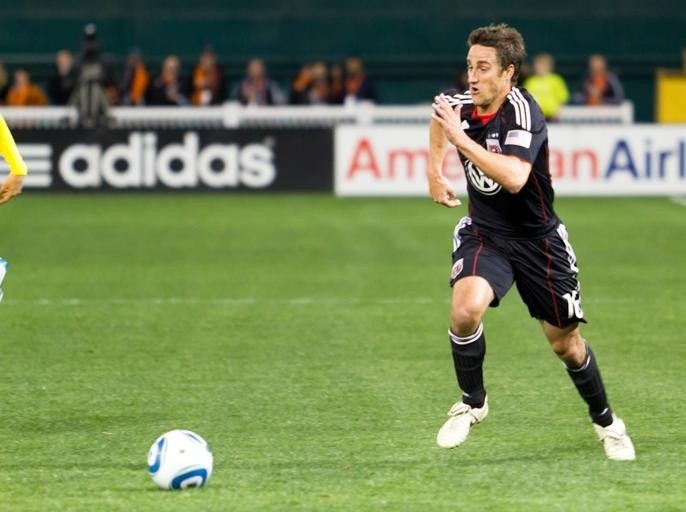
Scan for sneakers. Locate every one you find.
[436,394,488,449]
[592,412,635,461]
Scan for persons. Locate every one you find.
[1,23,379,108]
[575,52,625,107]
[424,23,639,462]
[520,51,572,123]
[0,107,28,302]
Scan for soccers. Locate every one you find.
[147,429,215,491]
